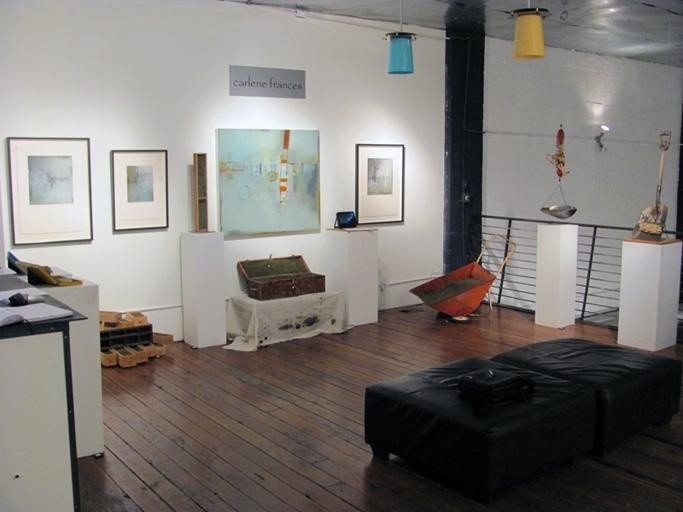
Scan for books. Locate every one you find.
[0,302,73,327]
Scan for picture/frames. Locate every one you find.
[356,143,406,225]
[111,149,169,232]
[7,137,93,246]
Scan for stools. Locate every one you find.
[364,356,595,505]
[489,339,682,445]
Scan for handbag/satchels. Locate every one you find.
[459,367,534,419]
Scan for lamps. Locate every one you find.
[386,0,416,74]
[510,0,549,58]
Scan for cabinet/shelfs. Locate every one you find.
[0,274,88,512]
